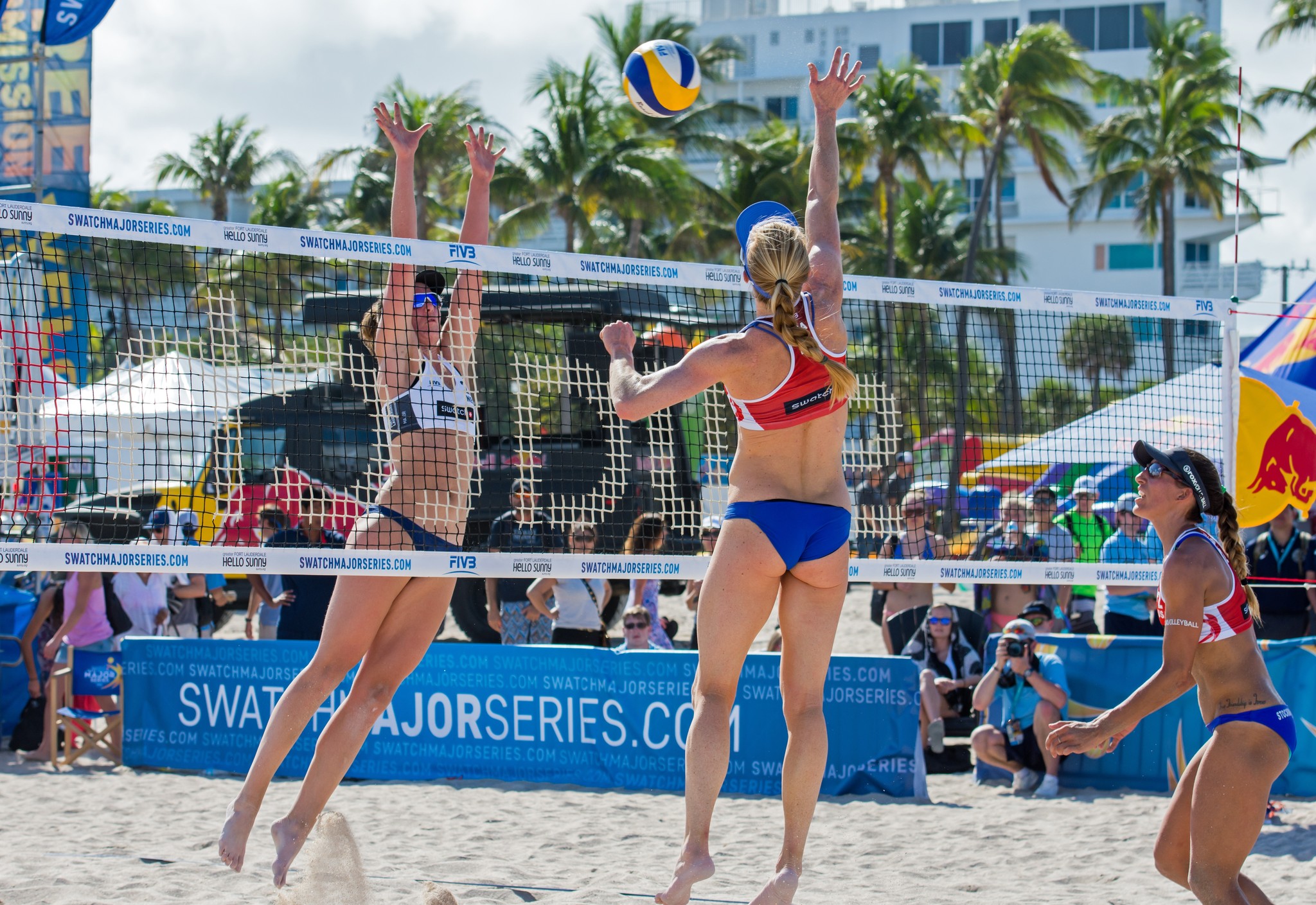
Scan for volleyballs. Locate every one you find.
[619,35,704,118]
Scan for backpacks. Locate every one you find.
[7,695,64,753]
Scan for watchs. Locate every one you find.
[1023,666,1035,678]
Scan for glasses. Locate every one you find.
[1002,628,1026,635]
[1031,498,1055,506]
[903,508,926,517]
[701,530,720,538]
[1144,462,1205,497]
[1075,493,1094,497]
[1026,617,1050,626]
[574,535,594,543]
[625,622,647,630]
[148,529,163,533]
[413,293,444,308]
[514,490,533,498]
[928,616,951,625]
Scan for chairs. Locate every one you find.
[50,645,123,765]
[887,604,990,738]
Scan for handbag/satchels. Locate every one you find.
[871,533,898,625]
[104,589,133,636]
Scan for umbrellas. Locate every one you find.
[966,275,1316,498]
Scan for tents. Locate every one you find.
[0,351,331,494]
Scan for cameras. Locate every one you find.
[1005,640,1031,659]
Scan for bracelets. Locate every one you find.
[992,664,1002,673]
[245,618,251,622]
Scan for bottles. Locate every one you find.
[1003,519,1018,549]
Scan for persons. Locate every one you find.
[17,505,238,765]
[969,618,1072,799]
[485,478,563,647]
[601,47,867,905]
[617,513,721,651]
[1053,475,1115,634]
[245,503,287,640]
[872,492,957,656]
[966,488,1075,635]
[247,487,348,640]
[855,450,915,559]
[218,101,505,892]
[901,602,984,754]
[1099,491,1166,638]
[526,515,612,649]
[1243,503,1315,640]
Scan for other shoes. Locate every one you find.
[926,719,944,754]
[1009,769,1059,798]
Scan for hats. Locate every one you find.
[142,508,199,530]
[896,451,913,463]
[735,201,799,300]
[998,601,1052,644]
[415,269,445,295]
[698,516,723,528]
[1133,440,1210,512]
[1072,475,1140,512]
[510,477,538,494]
